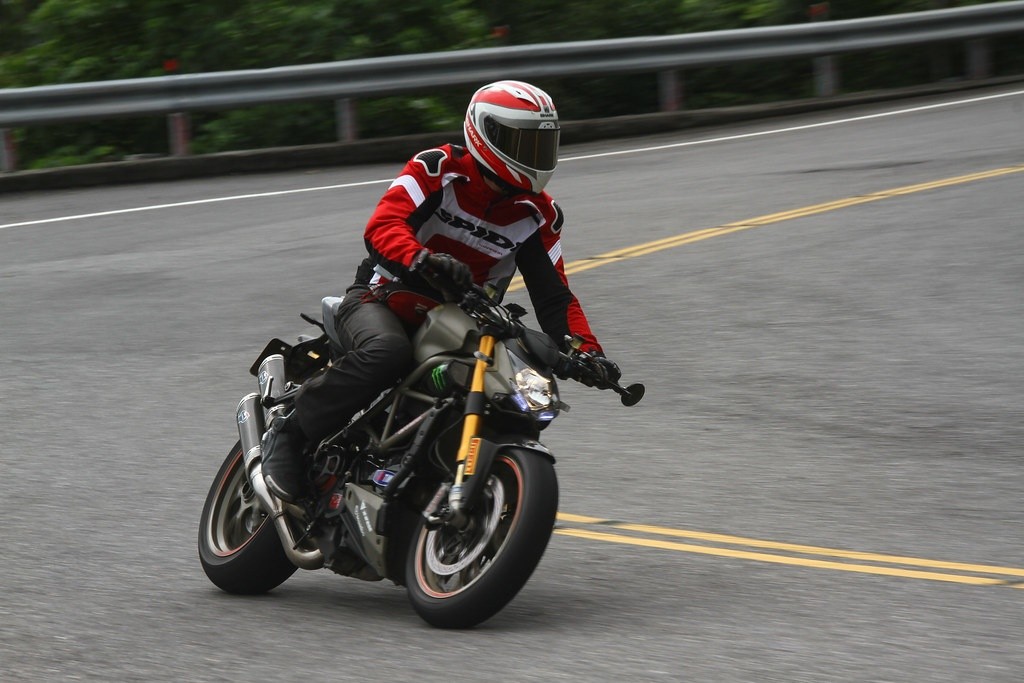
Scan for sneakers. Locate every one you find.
[259,415,310,503]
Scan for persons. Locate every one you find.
[263,80,621,504]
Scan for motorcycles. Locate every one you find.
[198,255,646,632]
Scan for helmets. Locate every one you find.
[462,80,561,193]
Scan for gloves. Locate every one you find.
[414,250,473,302]
[579,350,621,390]
